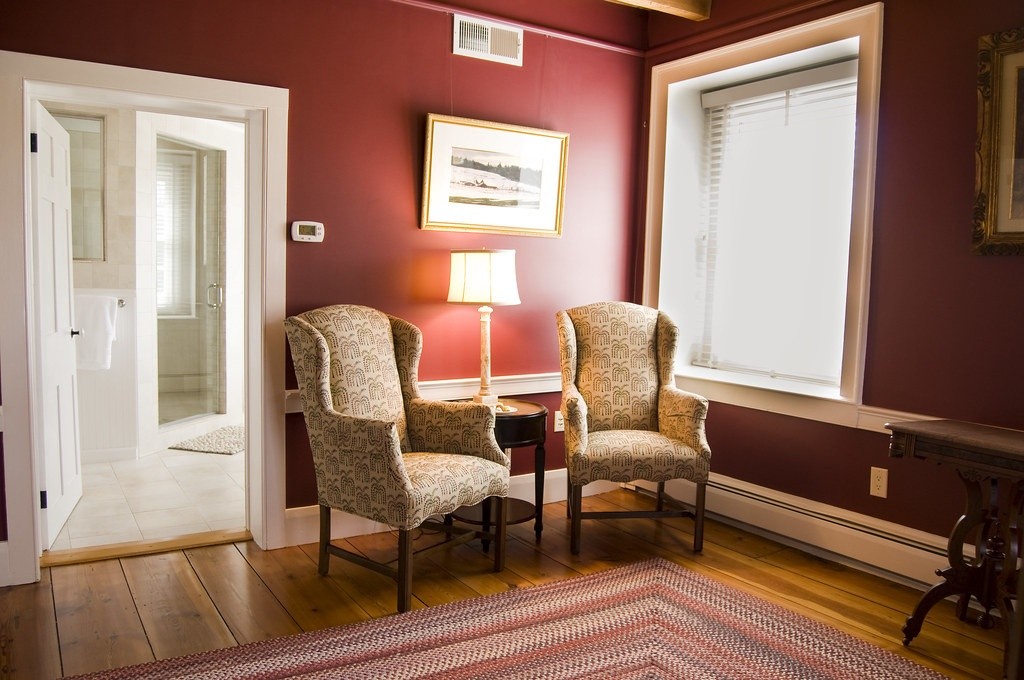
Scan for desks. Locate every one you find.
[884,419,1024,680]
[443,397,549,572]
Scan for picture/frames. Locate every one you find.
[972,26,1024,257]
[418,111,570,237]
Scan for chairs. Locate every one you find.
[282,303,512,613]
[555,300,712,555]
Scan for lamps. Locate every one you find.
[43,108,107,262]
[447,246,521,413]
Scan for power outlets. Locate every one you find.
[869,466,888,498]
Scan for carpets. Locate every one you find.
[168,424,244,455]
[53,558,954,679]
